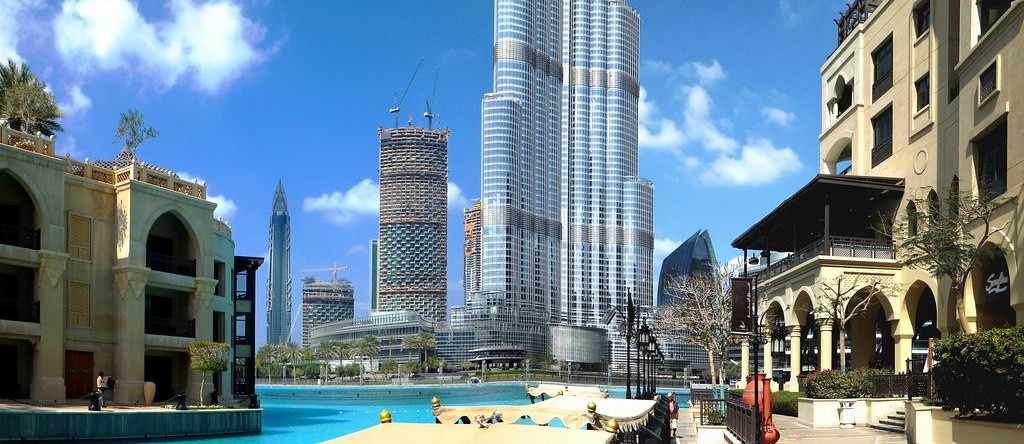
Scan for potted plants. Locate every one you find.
[704,407,724,425]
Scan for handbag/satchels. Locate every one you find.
[673,401,679,420]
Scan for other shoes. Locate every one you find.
[102,405,107,408]
[673,434,676,436]
[671,436,674,439]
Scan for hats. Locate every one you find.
[667,393,672,397]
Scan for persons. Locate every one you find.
[668,395,678,439]
[97,371,111,407]
